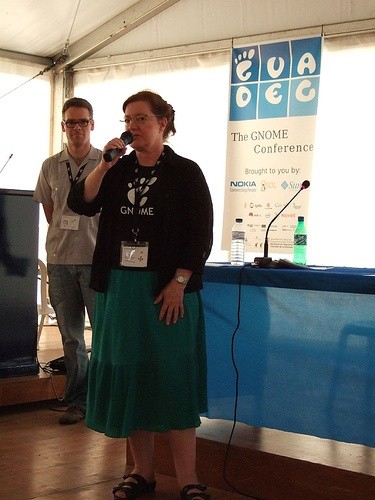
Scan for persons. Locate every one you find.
[33,97,105,425]
[67,91,214,500]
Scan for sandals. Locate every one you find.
[112,472,157,500]
[180,483,210,500]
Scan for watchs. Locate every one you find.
[173,273,188,285]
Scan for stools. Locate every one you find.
[326,320,375,417]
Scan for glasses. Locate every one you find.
[62,118,91,128]
[124,114,163,126]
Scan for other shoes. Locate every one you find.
[59,407,87,424]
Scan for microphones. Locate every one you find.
[0,153,14,172]
[102,130,135,163]
[254,180,311,268]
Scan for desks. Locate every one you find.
[127,262,375,500]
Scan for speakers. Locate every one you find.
[0,189,40,377]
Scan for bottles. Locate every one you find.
[293,216,306,265]
[231,218,246,266]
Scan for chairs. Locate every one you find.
[37,259,54,351]
